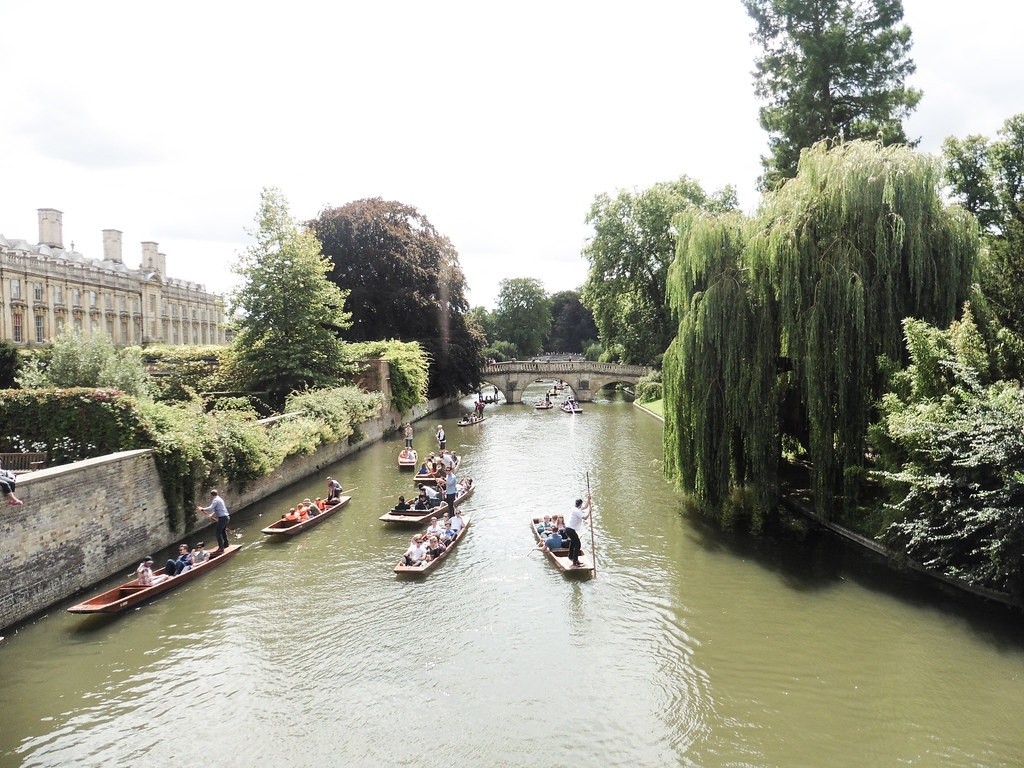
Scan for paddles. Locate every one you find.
[423,461,446,493]
[586,471,597,581]
[323,487,359,501]
[185,498,234,534]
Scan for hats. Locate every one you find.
[144,556,154,564]
[303,499,311,503]
[429,452,434,457]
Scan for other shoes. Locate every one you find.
[8,501,16,507]
[574,562,583,565]
[224,544,229,548]
[213,549,224,554]
[12,501,22,506]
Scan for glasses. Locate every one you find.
[197,546,202,549]
[417,542,422,543]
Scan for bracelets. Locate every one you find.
[201,508,203,511]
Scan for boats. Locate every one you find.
[480,398,501,403]
[535,385,565,409]
[67,544,243,614]
[531,517,594,573]
[379,485,476,525]
[558,403,584,413]
[413,455,462,484]
[457,416,487,426]
[393,515,472,576]
[261,496,352,536]
[398,449,419,470]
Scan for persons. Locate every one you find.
[282,476,343,522]
[404,423,413,450]
[199,489,230,553]
[137,541,210,587]
[0,475,24,505]
[462,378,580,424]
[531,515,572,552]
[395,449,473,568]
[565,495,592,566]
[436,424,446,450]
